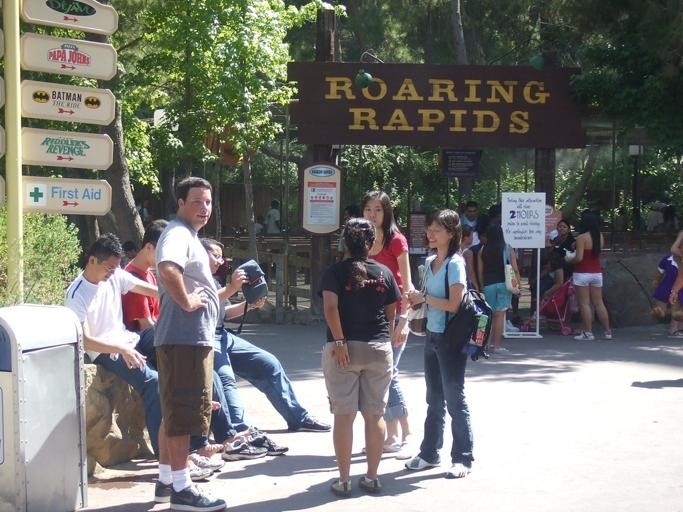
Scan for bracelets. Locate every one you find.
[423,295,426,303]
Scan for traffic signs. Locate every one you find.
[21,176,112,216]
[22,80,116,127]
[19,31,120,81]
[21,0,119,36]
[22,128,114,170]
[443,150,481,178]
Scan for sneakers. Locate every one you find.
[359,476,381,492]
[296,415,331,432]
[405,455,440,469]
[601,330,613,340]
[169,485,226,511]
[574,331,595,341]
[513,316,524,324]
[397,441,412,459]
[363,443,401,453]
[154,481,175,502]
[494,348,511,356]
[188,425,224,480]
[669,327,683,338]
[332,479,352,496]
[445,463,471,477]
[504,319,519,331]
[224,426,289,458]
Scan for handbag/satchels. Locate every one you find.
[237,259,268,304]
[444,257,492,361]
[408,294,427,336]
[505,263,520,296]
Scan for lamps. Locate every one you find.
[354,50,386,87]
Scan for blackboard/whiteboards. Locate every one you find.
[501,192,546,249]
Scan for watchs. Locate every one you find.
[336,336,347,346]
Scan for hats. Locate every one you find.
[549,229,559,240]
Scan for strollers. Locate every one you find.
[521,277,575,334]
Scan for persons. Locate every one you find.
[460,195,682,355]
[337,206,360,262]
[319,218,401,497]
[154,179,226,510]
[362,191,416,459]
[68,223,330,479]
[255,199,283,235]
[404,208,475,478]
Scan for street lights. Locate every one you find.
[624,126,646,207]
[356,51,411,246]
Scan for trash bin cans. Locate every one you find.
[0,304,88,512]
